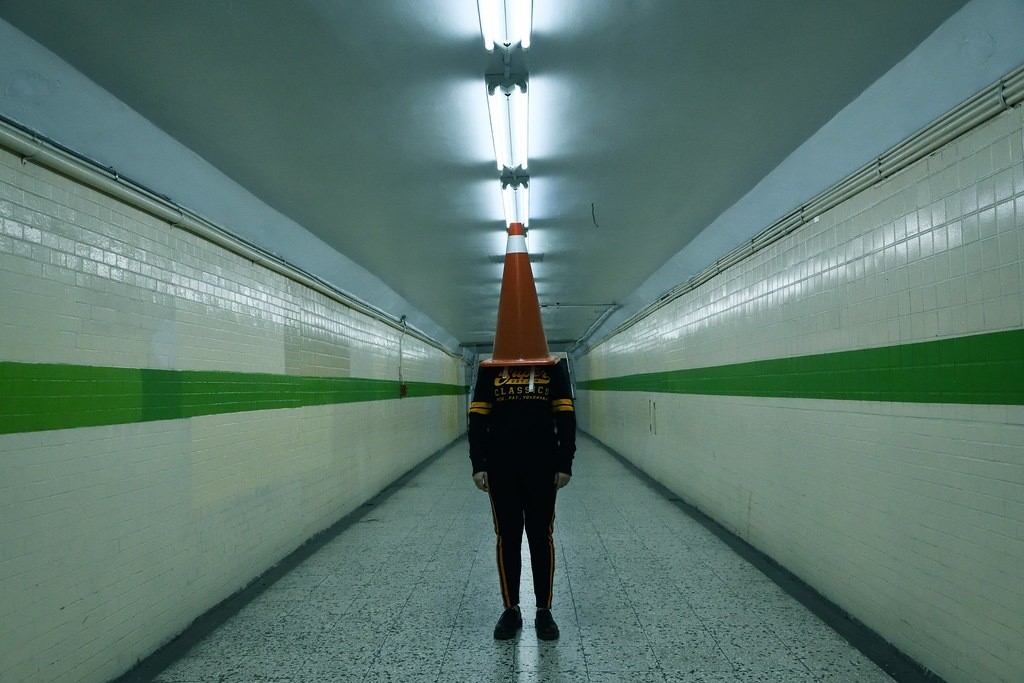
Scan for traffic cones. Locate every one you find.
[484,223,561,367]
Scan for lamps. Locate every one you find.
[484,71,531,174]
[501,176,529,238]
[476,0,533,53]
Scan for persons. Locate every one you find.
[468,357,578,641]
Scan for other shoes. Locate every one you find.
[494,607,523,639]
[535,609,560,640]
